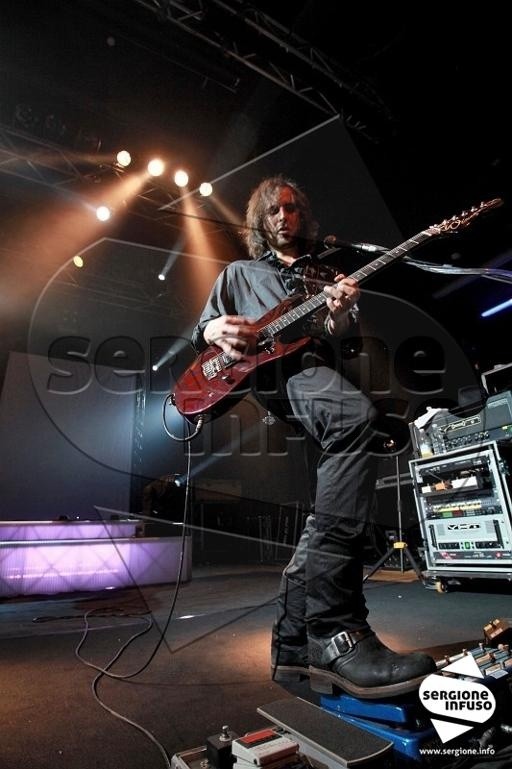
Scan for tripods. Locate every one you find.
[363,456,427,588]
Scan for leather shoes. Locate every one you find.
[268,605,312,684]
[306,608,445,703]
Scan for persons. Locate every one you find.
[190,174,438,700]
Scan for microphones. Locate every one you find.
[322,235,377,256]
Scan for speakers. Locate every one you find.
[200,500,251,561]
[275,500,300,561]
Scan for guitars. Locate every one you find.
[172,196,504,423]
